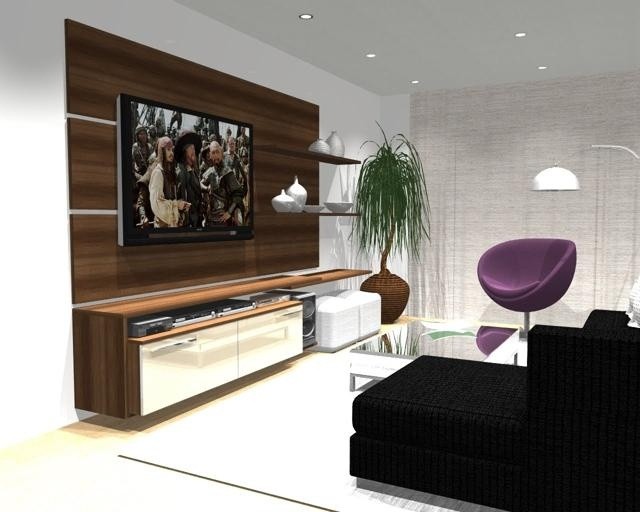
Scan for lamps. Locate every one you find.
[527,142,639,328]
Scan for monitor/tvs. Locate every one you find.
[116,93,254,248]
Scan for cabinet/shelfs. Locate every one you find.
[252,143,365,220]
[135,322,241,419]
[242,304,302,388]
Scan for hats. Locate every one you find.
[174,129,202,163]
[135,124,148,134]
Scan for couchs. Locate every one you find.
[347,307,636,508]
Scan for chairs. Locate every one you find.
[478,231,578,335]
[472,323,512,358]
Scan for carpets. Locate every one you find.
[117,352,511,511]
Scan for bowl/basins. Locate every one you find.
[301,204,324,213]
[324,202,354,213]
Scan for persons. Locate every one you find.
[131,101,250,229]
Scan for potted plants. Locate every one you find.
[349,116,432,324]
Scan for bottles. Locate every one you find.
[308,130,345,158]
[271,176,308,213]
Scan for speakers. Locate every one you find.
[266,289,316,348]
